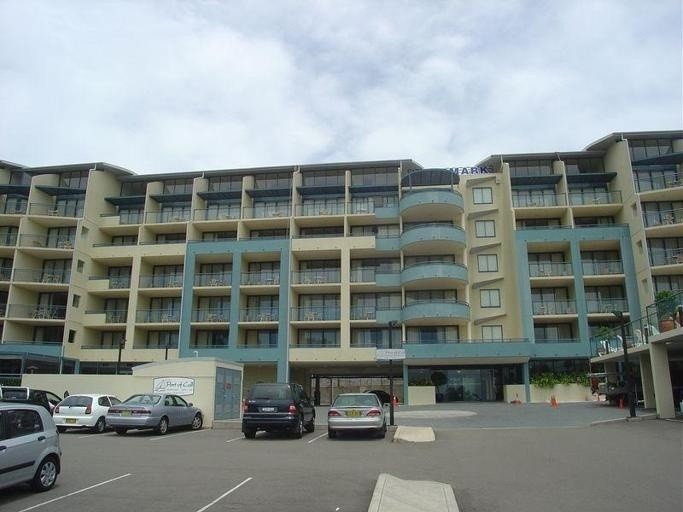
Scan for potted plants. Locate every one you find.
[653,289,678,332]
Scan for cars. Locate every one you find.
[242,382,398,439]
[0,386,203,492]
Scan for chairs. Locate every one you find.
[598,324,659,357]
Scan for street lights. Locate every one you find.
[611,310,636,418]
